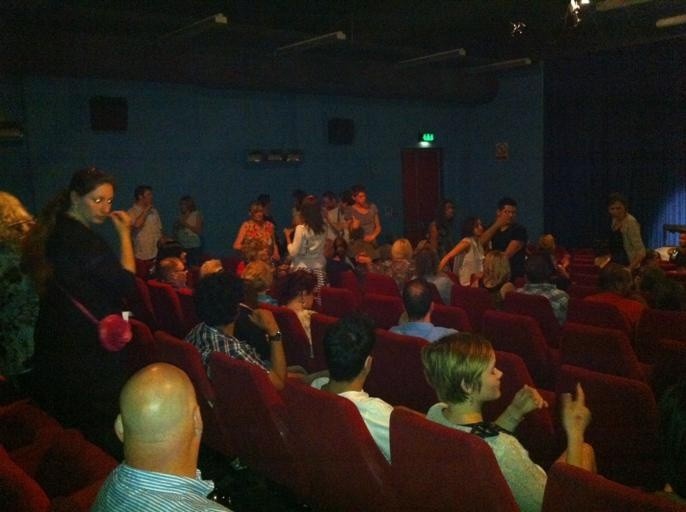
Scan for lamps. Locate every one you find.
[276,30,348,56]
[399,47,466,67]
[563,0,590,29]
[147,12,228,48]
[469,56,532,76]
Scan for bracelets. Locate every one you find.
[505,407,525,423]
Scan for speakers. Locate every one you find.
[327,118,353,146]
[90,96,128,132]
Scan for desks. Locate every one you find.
[663,224,686,245]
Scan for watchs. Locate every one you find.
[265,330,281,344]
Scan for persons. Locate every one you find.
[0,189,41,401]
[150,184,686,334]
[654,376,686,509]
[420,330,598,512]
[18,165,133,441]
[87,361,238,512]
[126,184,165,284]
[180,270,288,393]
[309,314,396,468]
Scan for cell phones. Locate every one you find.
[238,302,254,316]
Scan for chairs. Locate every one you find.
[0,375,119,512]
